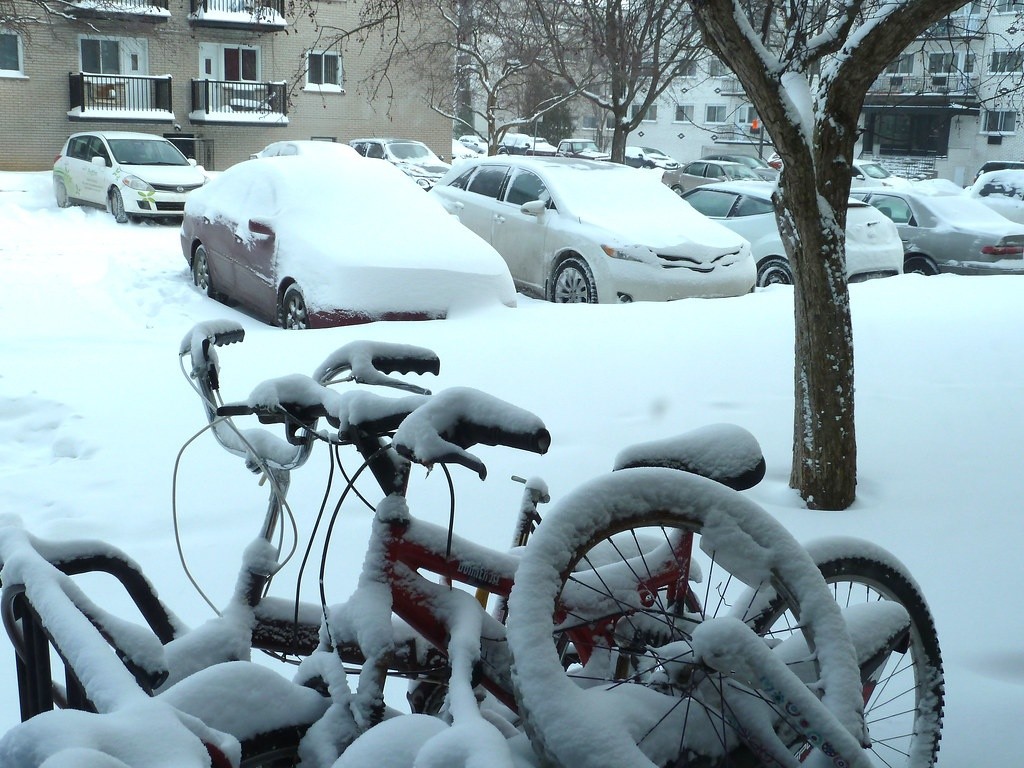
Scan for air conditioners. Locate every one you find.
[90,82,115,99]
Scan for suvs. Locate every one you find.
[555,138,611,162]
[350,137,453,192]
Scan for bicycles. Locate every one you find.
[161,317,948,768]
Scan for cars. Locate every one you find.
[53,132,208,223]
[180,153,520,332]
[625,147,681,171]
[677,179,903,288]
[969,161,1024,226]
[249,141,361,162]
[430,150,758,305]
[458,136,488,154]
[698,154,779,182]
[849,187,1023,275]
[660,160,765,195]
[851,159,907,188]
